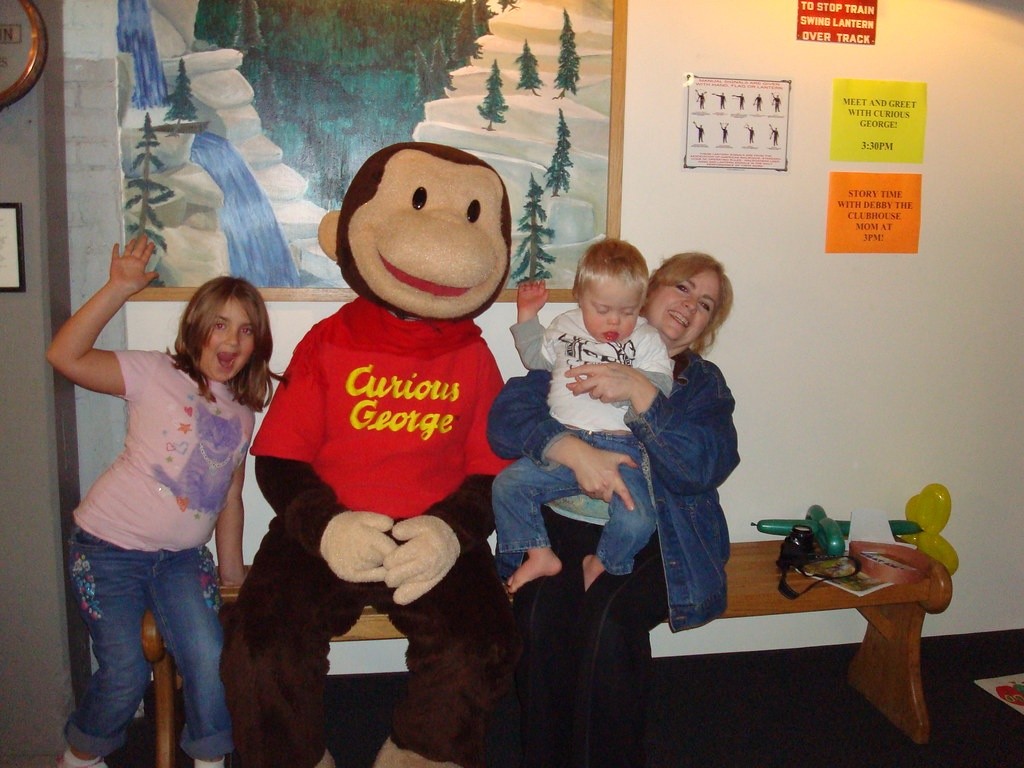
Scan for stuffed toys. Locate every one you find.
[217,142,525,768]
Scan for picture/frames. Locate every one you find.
[114,0,619,302]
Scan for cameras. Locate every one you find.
[778,525,819,570]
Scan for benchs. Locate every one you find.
[142,540,952,768]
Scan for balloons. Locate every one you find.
[751,483,959,576]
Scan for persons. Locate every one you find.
[44,234,288,768]
[486,237,740,768]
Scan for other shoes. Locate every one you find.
[56,752,109,768]
